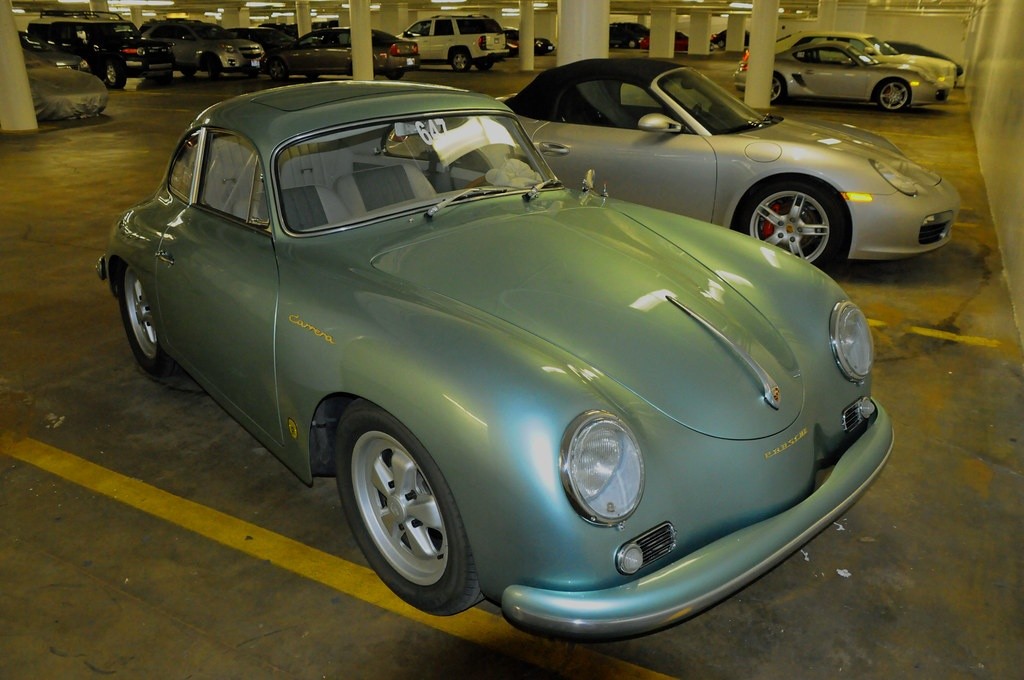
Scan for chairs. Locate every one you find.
[232,184,350,233]
[336,163,438,218]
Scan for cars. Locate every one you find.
[730,41,952,113]
[17,30,93,75]
[503,28,556,56]
[21,48,109,123]
[226,27,296,75]
[93,80,893,644]
[609,23,649,49]
[710,30,750,50]
[261,27,420,82]
[638,32,714,52]
[883,40,964,79]
[739,32,959,88]
[492,56,962,284]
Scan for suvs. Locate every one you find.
[396,14,510,73]
[139,18,266,82]
[25,10,176,90]
